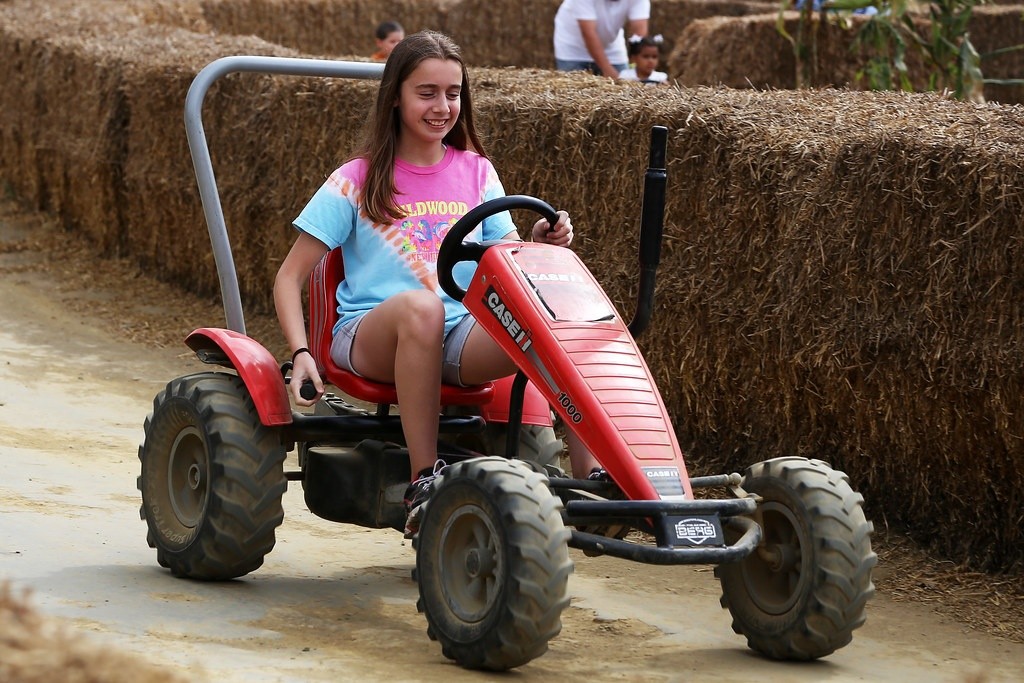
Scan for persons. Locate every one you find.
[372,22,404,59]
[618,34,670,86]
[554,0,651,79]
[273,30,631,557]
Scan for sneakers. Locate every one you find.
[404,458,450,539]
[581,468,630,557]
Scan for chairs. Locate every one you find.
[309,250,494,417]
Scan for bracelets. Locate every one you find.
[292,348,312,364]
